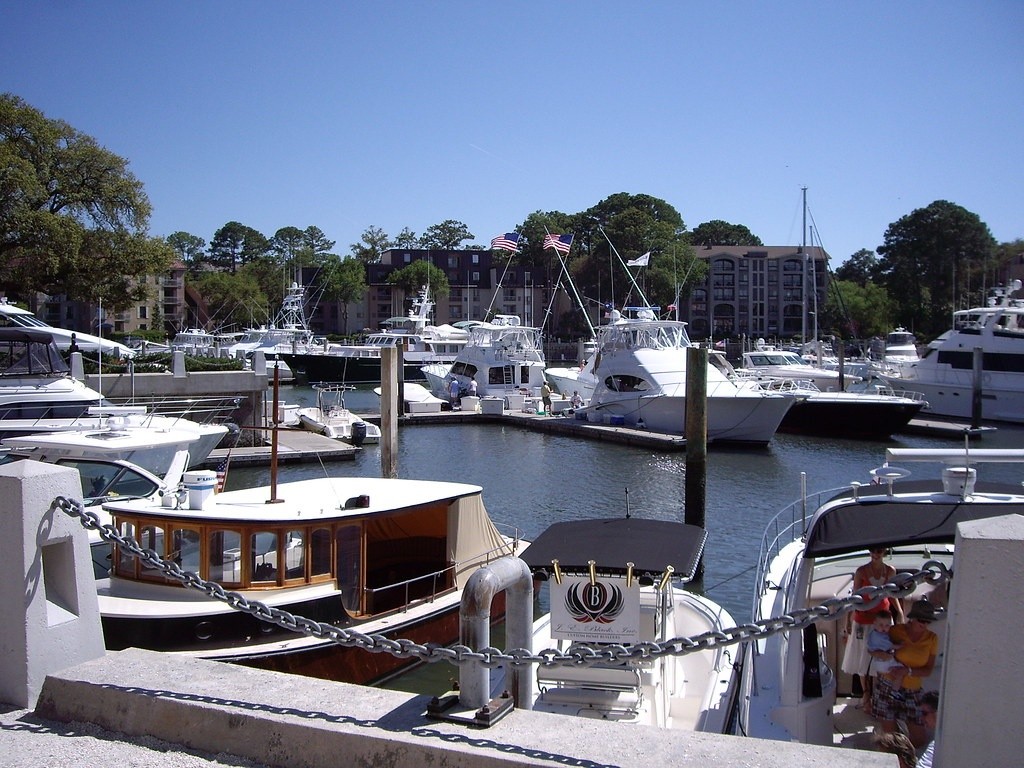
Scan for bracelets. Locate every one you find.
[908,666,912,677]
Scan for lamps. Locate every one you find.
[659,565,674,590]
[588,560,597,587]
[551,559,562,585]
[626,562,635,588]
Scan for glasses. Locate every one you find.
[915,617,932,624]
[918,710,934,717]
[870,548,884,554]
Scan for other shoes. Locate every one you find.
[550,414,554,417]
[863,697,872,715]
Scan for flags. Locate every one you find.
[491,232,519,252]
[543,233,574,253]
[626,251,650,267]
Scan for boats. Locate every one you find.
[1,185,1023,768]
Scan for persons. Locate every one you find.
[579,359,585,371]
[842,545,940,768]
[540,381,555,417]
[570,390,583,408]
[448,376,477,406]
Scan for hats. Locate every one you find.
[906,600,938,621]
[543,380,549,383]
